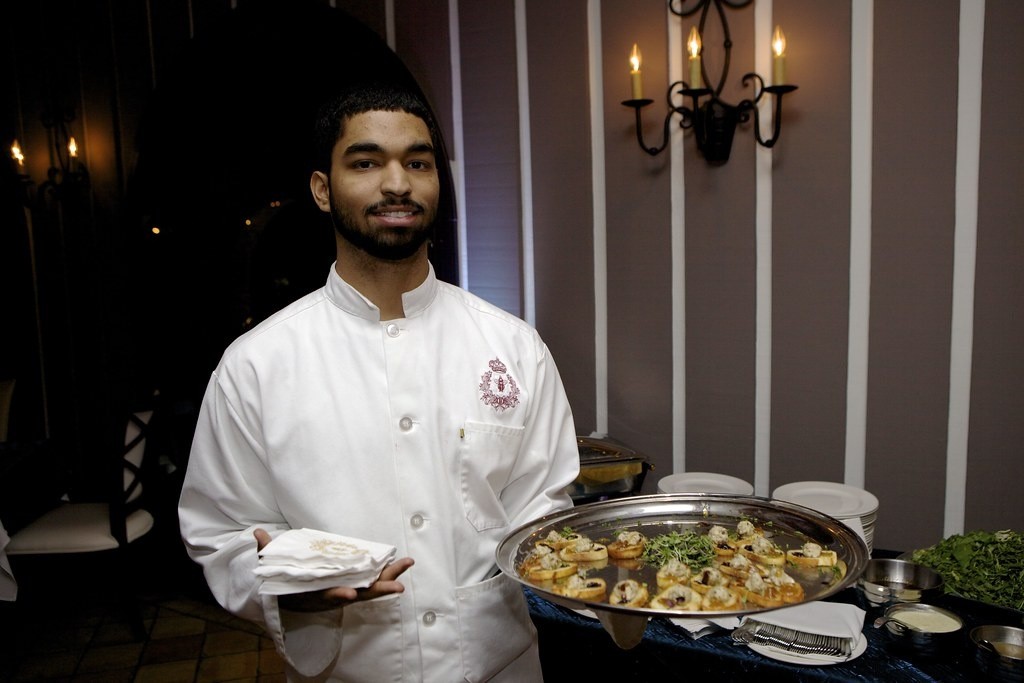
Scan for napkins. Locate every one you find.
[739,601,867,663]
[252,527,398,596]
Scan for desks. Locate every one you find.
[523,547,1024,683]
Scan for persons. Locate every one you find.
[177,87,648,683]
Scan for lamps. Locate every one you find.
[8,96,94,212]
[619,0,799,167]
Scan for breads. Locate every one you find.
[522,531,837,611]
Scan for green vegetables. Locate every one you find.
[557,513,798,567]
[902,528,1024,612]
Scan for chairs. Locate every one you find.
[8,386,161,642]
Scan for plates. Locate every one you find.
[749,624,867,665]
[494,493,869,618]
[772,481,879,555]
[971,623,1024,664]
[856,558,946,607]
[655,470,754,497]
[884,602,963,642]
